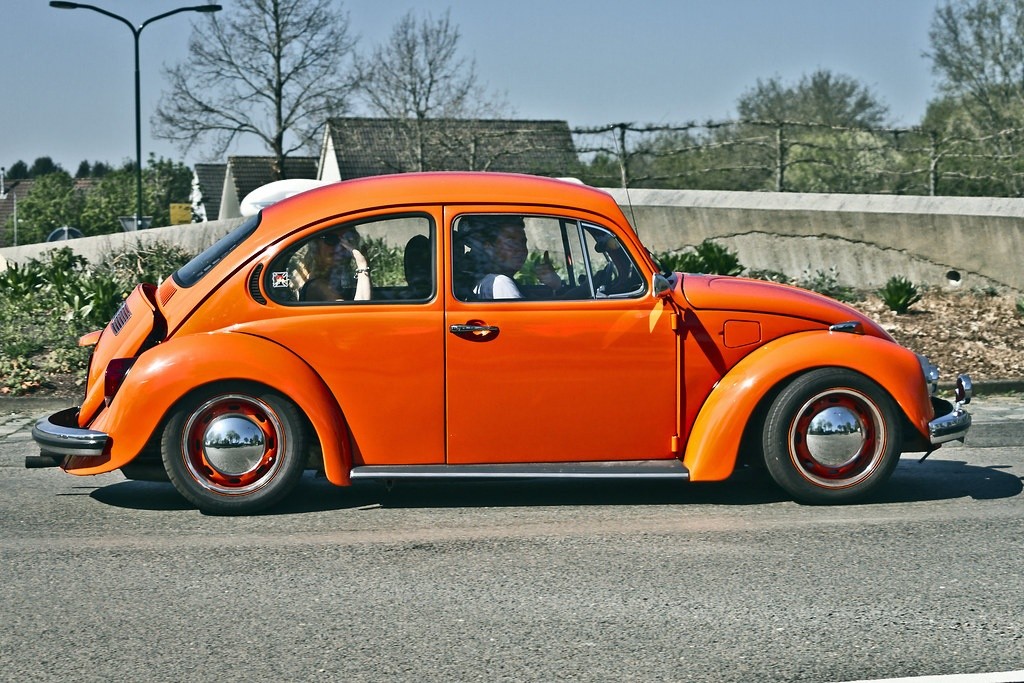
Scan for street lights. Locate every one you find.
[48,0,223,231]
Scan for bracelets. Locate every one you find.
[552,280,566,294]
[354,267,370,279]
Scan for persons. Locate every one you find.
[457,215,612,302]
[299,225,371,302]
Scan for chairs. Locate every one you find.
[402,229,471,301]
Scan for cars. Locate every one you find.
[22,171,972,518]
